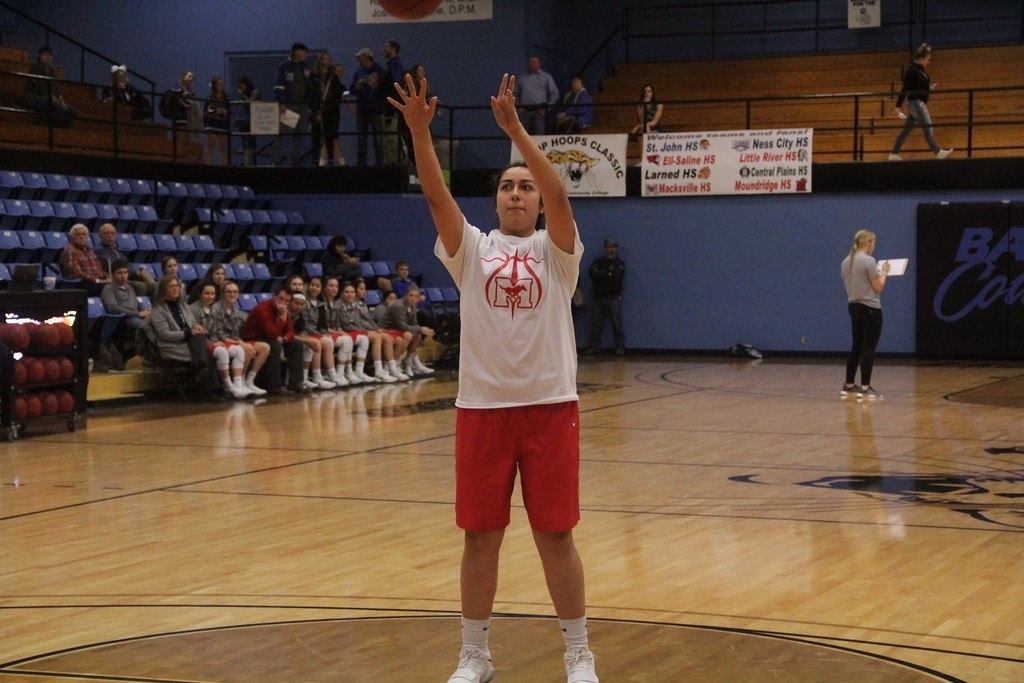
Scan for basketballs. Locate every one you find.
[0,323,74,349]
[377,0,443,21]
[15,390,73,419]
[14,356,74,385]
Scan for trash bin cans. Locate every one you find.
[433,136,460,193]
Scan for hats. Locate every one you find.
[354,48,373,57]
[604,238,618,247]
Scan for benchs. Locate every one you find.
[0,42,204,164]
[576,43,1024,162]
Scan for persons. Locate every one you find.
[273,39,439,169]
[232,76,261,165]
[102,249,436,402]
[554,76,592,134]
[101,64,151,121]
[840,230,891,398]
[631,83,664,167]
[571,274,587,325]
[514,55,559,135]
[95,223,155,297]
[584,239,627,354]
[59,223,112,296]
[202,77,231,165]
[22,46,77,128]
[322,235,350,281]
[387,72,599,683]
[888,43,953,160]
[169,71,198,164]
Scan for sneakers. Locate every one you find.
[841,384,859,395]
[563,645,599,683]
[856,386,883,397]
[448,647,494,683]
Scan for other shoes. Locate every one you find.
[207,393,226,402]
[586,347,600,354]
[889,154,903,160]
[225,386,267,400]
[287,383,311,393]
[298,378,434,404]
[303,355,434,388]
[226,399,266,417]
[936,149,954,160]
[615,346,624,355]
[267,385,288,395]
[192,367,209,383]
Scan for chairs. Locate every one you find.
[0,170,460,404]
